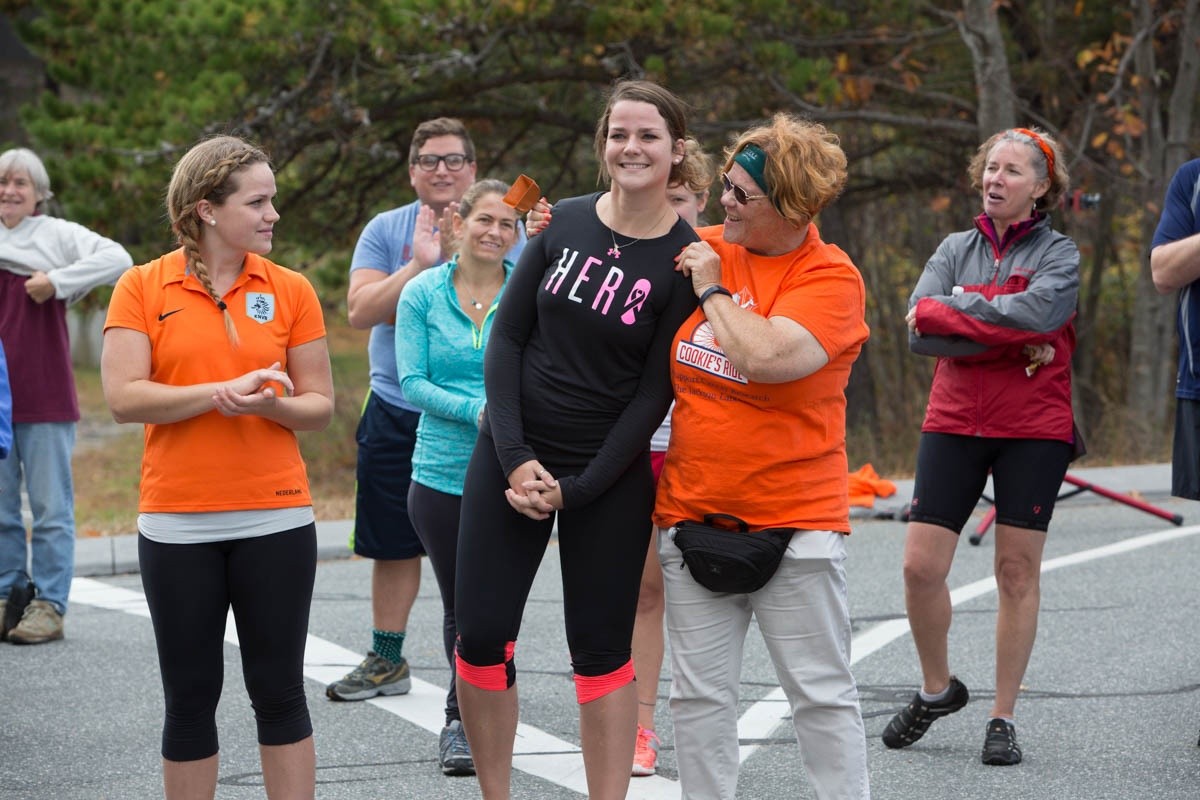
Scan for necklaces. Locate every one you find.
[457,262,500,310]
[608,202,670,251]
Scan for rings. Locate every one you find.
[539,469,546,476]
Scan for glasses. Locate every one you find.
[723,173,774,205]
[414,153,470,171]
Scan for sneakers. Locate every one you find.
[882,676,968,750]
[439,722,476,774]
[326,651,410,700]
[981,718,1021,764]
[632,723,660,774]
[0,596,32,639]
[8,600,65,643]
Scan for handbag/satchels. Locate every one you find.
[672,510,796,594]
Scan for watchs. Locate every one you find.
[698,286,730,312]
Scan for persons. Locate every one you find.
[453,80,715,800]
[1151,157,1200,501]
[879,129,1082,766]
[326,119,712,775]
[1,147,134,642]
[526,114,871,799]
[99,135,335,800]
